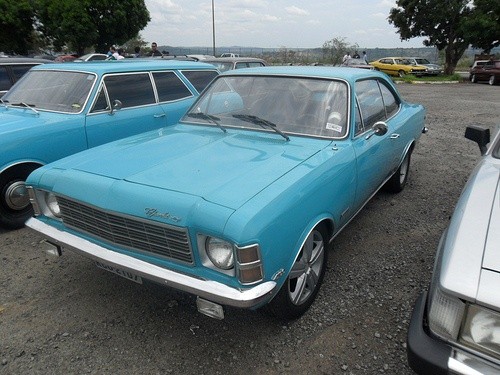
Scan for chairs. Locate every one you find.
[314,95,347,132]
[265,88,299,132]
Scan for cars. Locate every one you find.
[0,60,246,228]
[405,125,500,375]
[468,54,500,85]
[0,57,62,99]
[340,59,376,70]
[369,56,428,78]
[33,52,240,63]
[26,66,428,319]
[406,57,442,76]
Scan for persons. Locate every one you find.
[131,47,144,58]
[147,42,163,57]
[342,52,351,63]
[108,47,125,61]
[352,50,360,59]
[362,51,369,65]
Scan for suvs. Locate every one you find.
[196,56,270,73]
